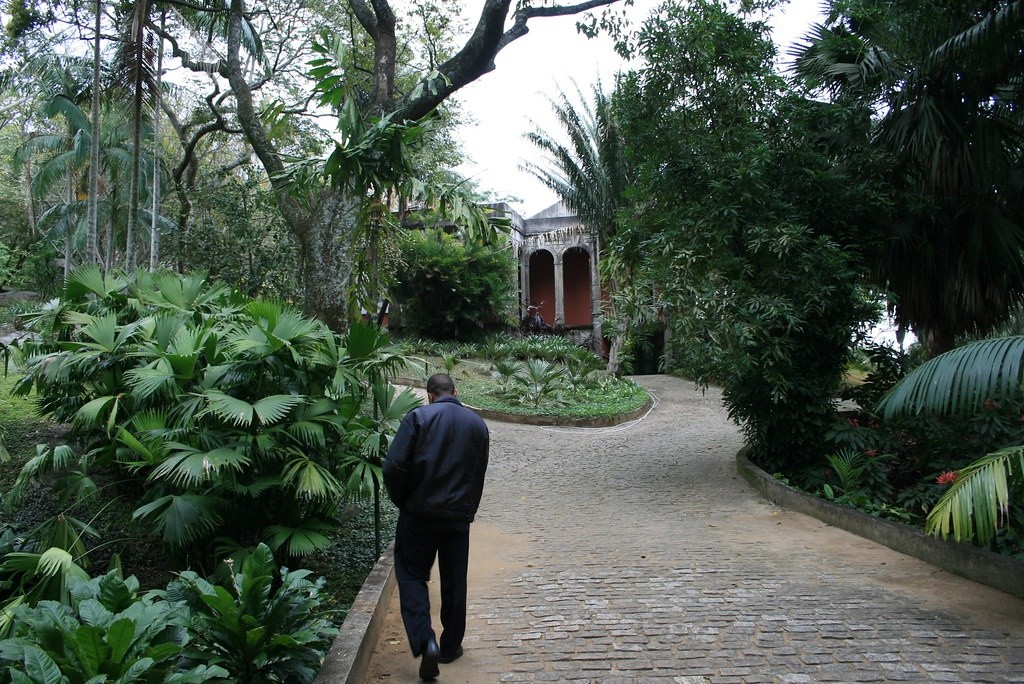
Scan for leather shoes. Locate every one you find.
[419,638,439,679]
[439,645,464,662]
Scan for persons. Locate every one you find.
[381,374,489,679]
